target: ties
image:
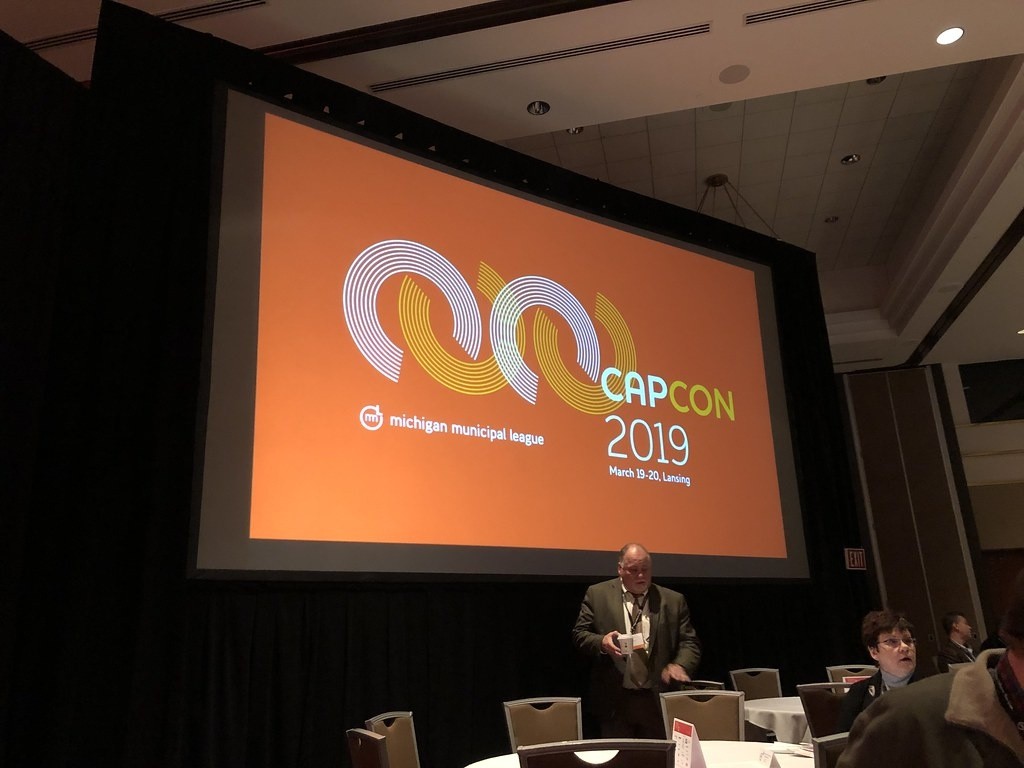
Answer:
[630,591,650,688]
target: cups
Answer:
[617,634,633,654]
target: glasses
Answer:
[877,637,917,647]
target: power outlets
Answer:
[928,633,934,642]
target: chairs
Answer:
[796,682,853,739]
[659,689,745,742]
[932,656,942,674]
[503,697,583,754]
[517,738,676,768]
[365,711,420,768]
[811,732,849,768]
[346,728,390,768]
[680,680,724,691]
[826,665,879,696]
[729,668,782,701]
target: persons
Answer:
[834,609,925,731]
[833,568,1024,768]
[936,612,986,673]
[571,540,700,739]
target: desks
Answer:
[463,739,816,768]
[743,696,812,744]
